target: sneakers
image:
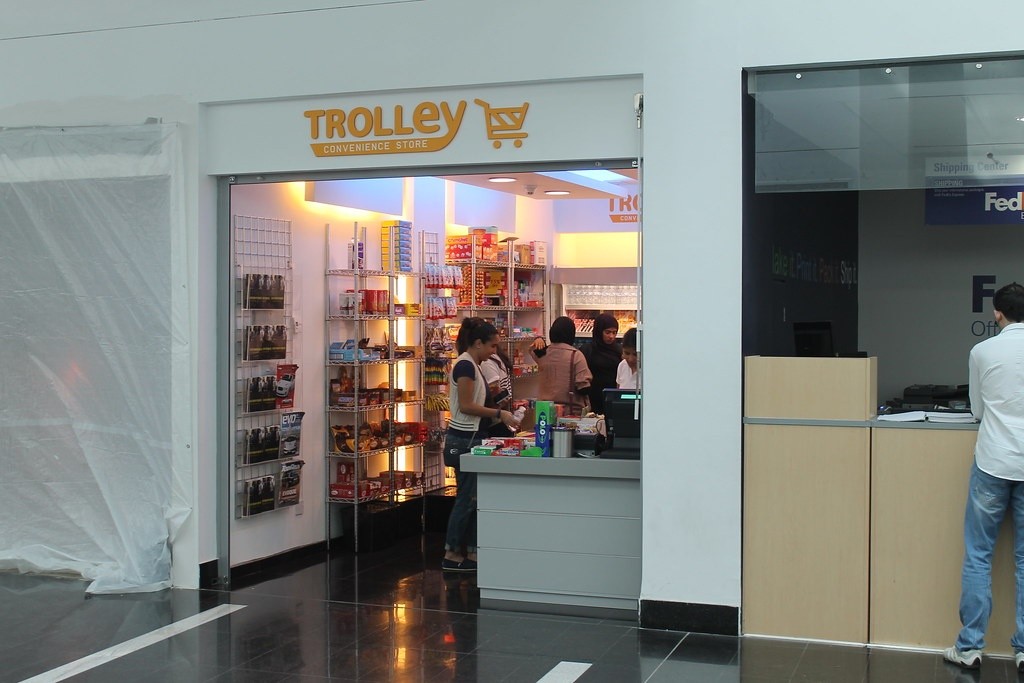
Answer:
[1016,652,1024,672]
[943,646,982,669]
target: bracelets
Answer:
[496,409,501,418]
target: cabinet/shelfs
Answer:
[565,303,638,339]
[320,222,428,562]
[417,229,511,496]
[512,243,547,435]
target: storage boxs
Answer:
[328,220,550,497]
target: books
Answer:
[877,411,976,423]
[243,273,306,517]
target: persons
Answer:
[941,282,1024,672]
[441,314,641,572]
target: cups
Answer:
[552,427,574,457]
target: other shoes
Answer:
[442,556,477,570]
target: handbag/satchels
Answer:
[443,443,469,467]
